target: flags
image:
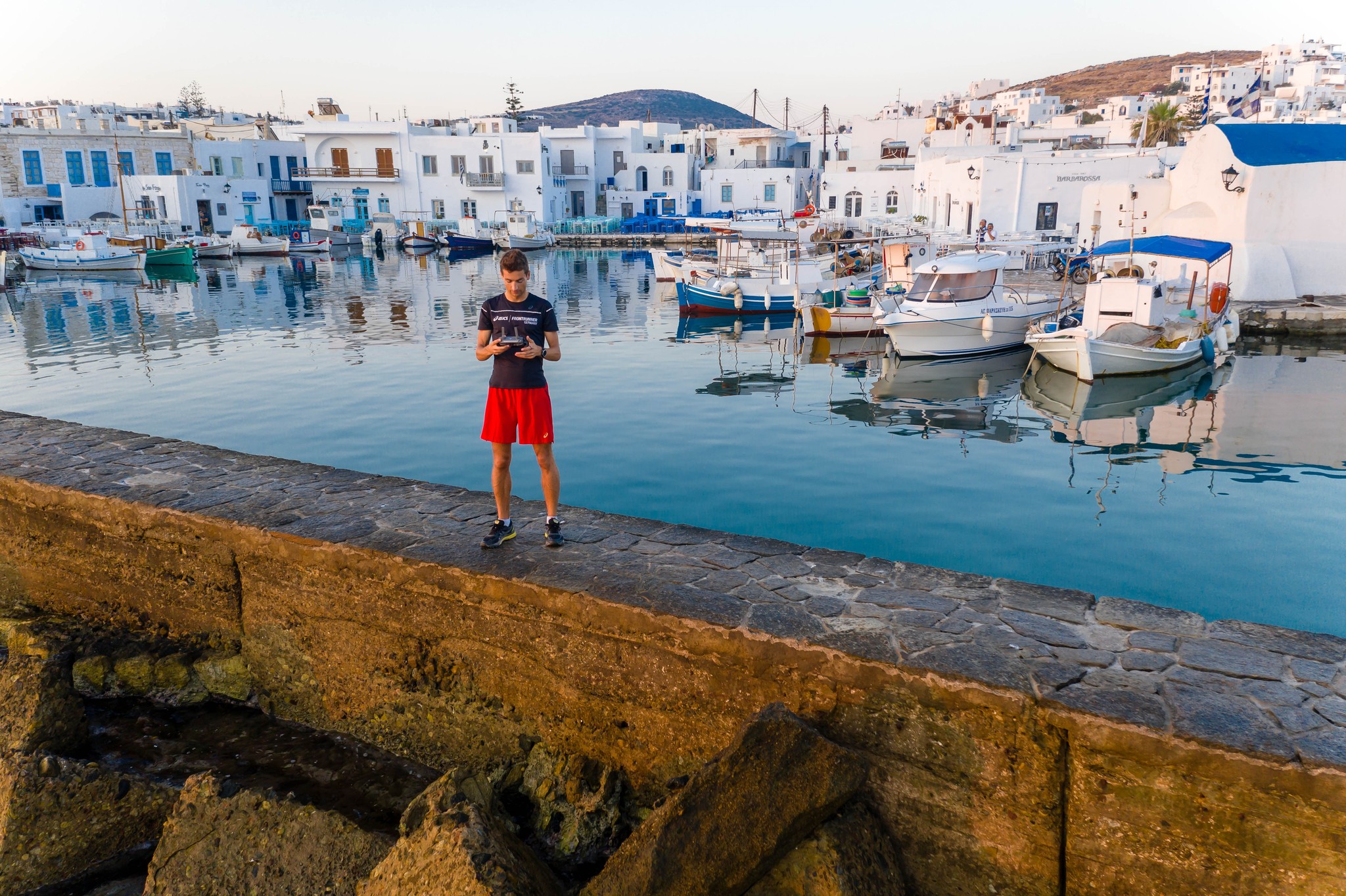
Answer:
[1226,73,1261,119]
[1200,69,1211,126]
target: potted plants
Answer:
[436,214,443,219]
[913,213,927,224]
[566,207,570,213]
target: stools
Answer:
[756,164,762,168]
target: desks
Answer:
[480,175,491,187]
[31,218,180,230]
[534,223,544,229]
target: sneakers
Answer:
[480,519,516,548]
[545,518,565,547]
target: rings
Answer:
[529,346,532,350]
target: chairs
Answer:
[618,185,625,191]
[555,249,717,269]
[253,219,311,236]
[820,215,1077,269]
[481,220,507,228]
[396,219,416,232]
[609,186,614,190]
[555,212,729,234]
[342,219,373,233]
[0,231,44,252]
[469,172,501,186]
[762,160,766,167]
[317,168,361,177]
[427,220,459,232]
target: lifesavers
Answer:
[805,205,815,215]
[180,236,188,238]
[464,216,474,218]
[76,242,84,250]
[293,232,300,239]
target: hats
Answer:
[199,211,203,213]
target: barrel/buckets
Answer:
[821,290,842,307]
[846,290,870,307]
[886,287,906,294]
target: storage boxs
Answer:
[247,231,262,241]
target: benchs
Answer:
[76,218,196,241]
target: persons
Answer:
[199,211,212,237]
[976,219,997,250]
[475,249,562,549]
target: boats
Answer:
[197,259,235,268]
[145,265,197,282]
[867,246,1077,361]
[1022,235,1241,385]
[493,197,552,252]
[648,178,887,318]
[799,337,894,364]
[444,216,496,250]
[399,211,435,247]
[796,233,938,338]
[233,256,293,269]
[674,317,804,344]
[868,354,1026,401]
[290,205,368,245]
[18,132,289,270]
[360,213,410,247]
[1018,362,1216,430]
[25,269,151,285]
[289,237,333,253]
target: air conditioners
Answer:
[182,168,193,176]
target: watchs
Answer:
[539,346,548,359]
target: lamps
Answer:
[380,193,384,197]
[223,182,232,194]
[483,140,488,152]
[650,196,669,200]
[689,196,691,200]
[536,185,567,200]
[967,165,980,180]
[854,187,855,189]
[203,189,206,193]
[887,209,895,214]
[892,184,926,193]
[1221,164,1245,193]
[726,174,790,183]
[259,197,261,201]
[932,265,938,273]
[613,196,628,200]
[822,180,827,190]
[334,192,337,197]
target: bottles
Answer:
[843,300,846,307]
[841,303,843,307]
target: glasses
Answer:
[988,223,993,225]
